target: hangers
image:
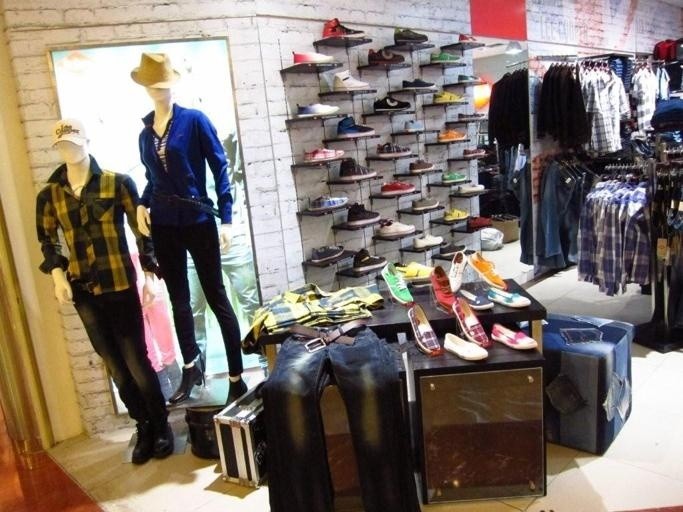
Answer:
[499,59,529,75]
[541,54,668,80]
[540,152,656,184]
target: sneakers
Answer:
[458,75,483,83]
[430,266,456,311]
[351,248,387,271]
[458,184,485,193]
[448,251,467,293]
[322,18,365,38]
[347,203,380,225]
[437,129,468,142]
[409,159,435,173]
[337,117,375,138]
[433,92,463,103]
[303,148,345,163]
[381,263,414,306]
[394,27,428,43]
[402,79,436,89]
[441,171,467,183]
[463,149,486,158]
[333,71,370,90]
[394,261,435,282]
[413,233,443,248]
[412,198,439,211]
[467,251,508,290]
[379,219,416,237]
[381,181,416,195]
[376,142,412,157]
[294,53,334,63]
[308,195,348,210]
[458,113,487,120]
[311,245,344,263]
[458,34,476,42]
[440,242,466,257]
[404,120,425,132]
[374,97,411,111]
[444,208,468,221]
[296,103,340,118]
[339,158,377,180]
[430,51,461,62]
[368,49,404,64]
[466,216,493,230]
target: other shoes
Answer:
[444,333,488,361]
[457,289,494,310]
[488,288,531,307]
[452,297,489,347]
[408,303,441,357]
[491,323,537,349]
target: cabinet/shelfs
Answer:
[263,340,410,504]
[405,335,553,505]
[258,276,550,472]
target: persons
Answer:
[123,213,182,404]
[185,160,268,386]
[129,53,248,407]
[34,117,175,466]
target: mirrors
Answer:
[468,32,535,283]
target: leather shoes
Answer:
[168,362,202,403]
[132,420,173,464]
[225,376,247,406]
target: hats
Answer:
[131,52,179,89]
[53,119,89,145]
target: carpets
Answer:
[44,226,682,512]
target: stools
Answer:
[530,311,634,457]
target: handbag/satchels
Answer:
[655,39,677,60]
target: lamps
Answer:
[503,40,525,56]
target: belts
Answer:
[291,319,366,352]
[152,191,221,217]
